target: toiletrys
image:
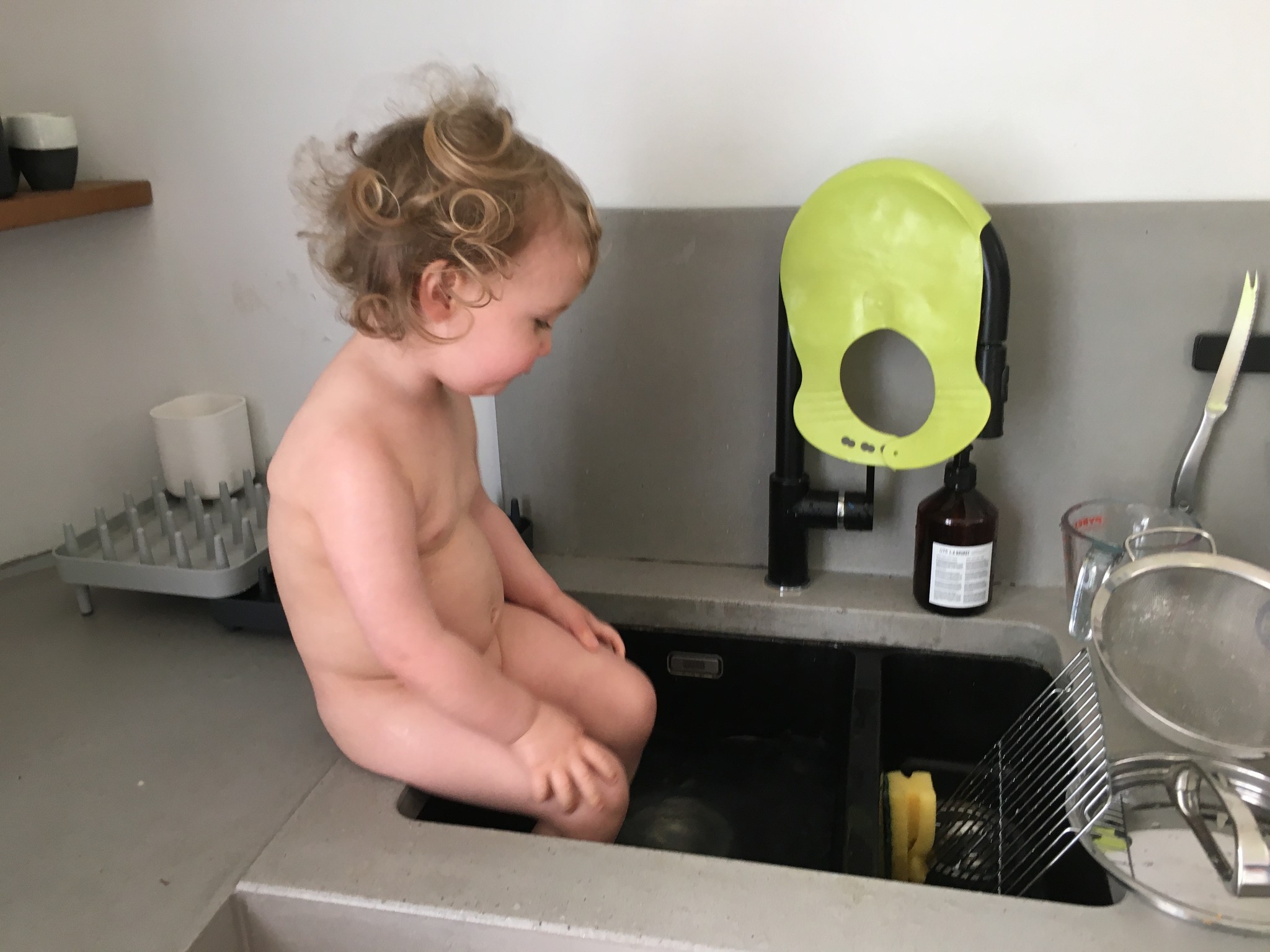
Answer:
[912,439,999,617]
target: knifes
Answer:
[1169,270,1262,518]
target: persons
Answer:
[264,59,660,845]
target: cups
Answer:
[1061,499,1203,639]
[150,392,256,500]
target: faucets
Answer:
[767,210,1013,595]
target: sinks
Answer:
[397,586,1137,907]
